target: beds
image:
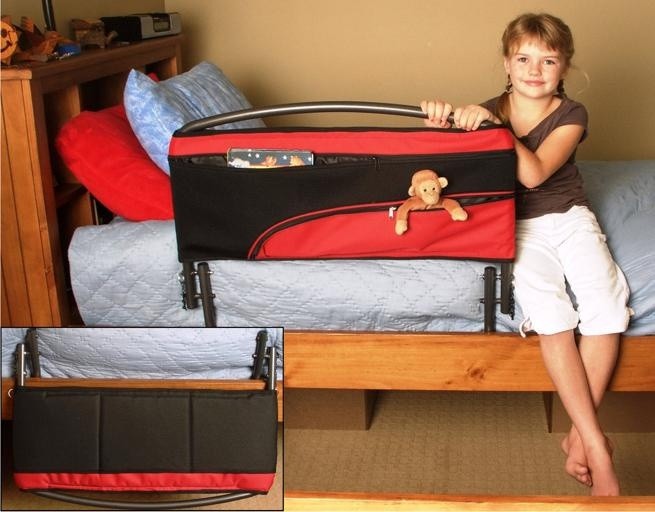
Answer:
[68,158,653,392]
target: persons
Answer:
[420,11,633,498]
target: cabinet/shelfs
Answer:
[0,32,189,326]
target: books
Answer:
[226,146,315,170]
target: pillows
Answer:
[58,60,266,220]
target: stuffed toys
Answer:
[393,168,469,236]
